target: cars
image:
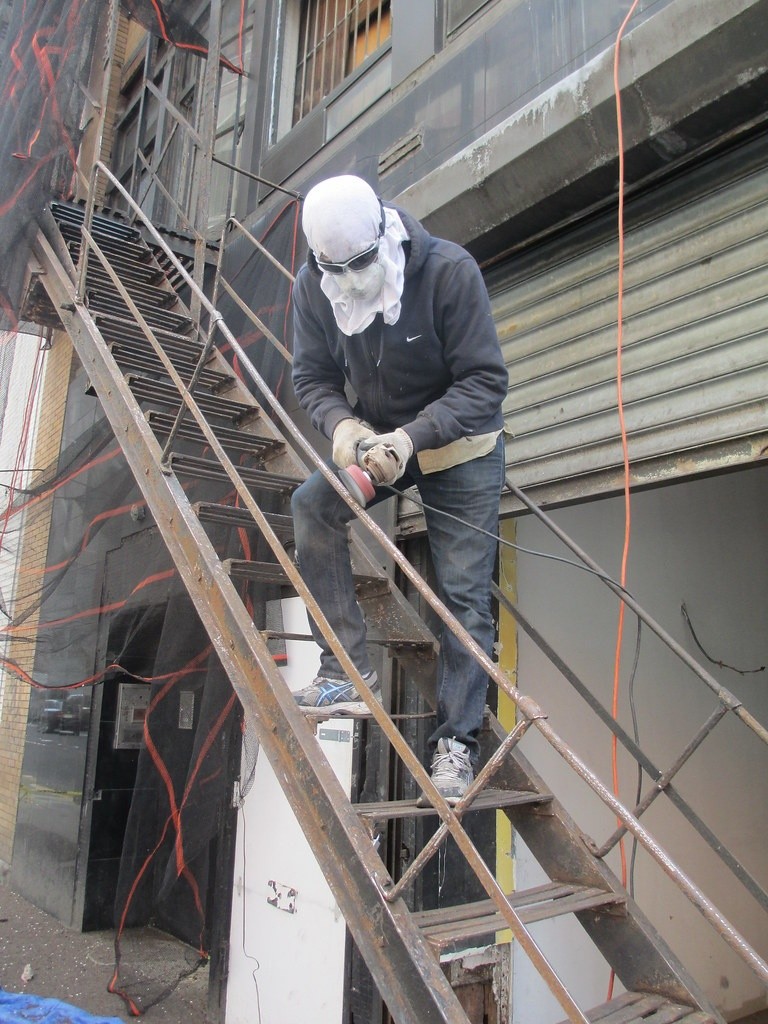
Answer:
[41,693,93,733]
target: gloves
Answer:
[332,418,376,469]
[359,428,414,485]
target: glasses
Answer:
[315,235,379,275]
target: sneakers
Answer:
[292,670,382,715]
[417,736,475,807]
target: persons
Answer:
[289,174,509,808]
